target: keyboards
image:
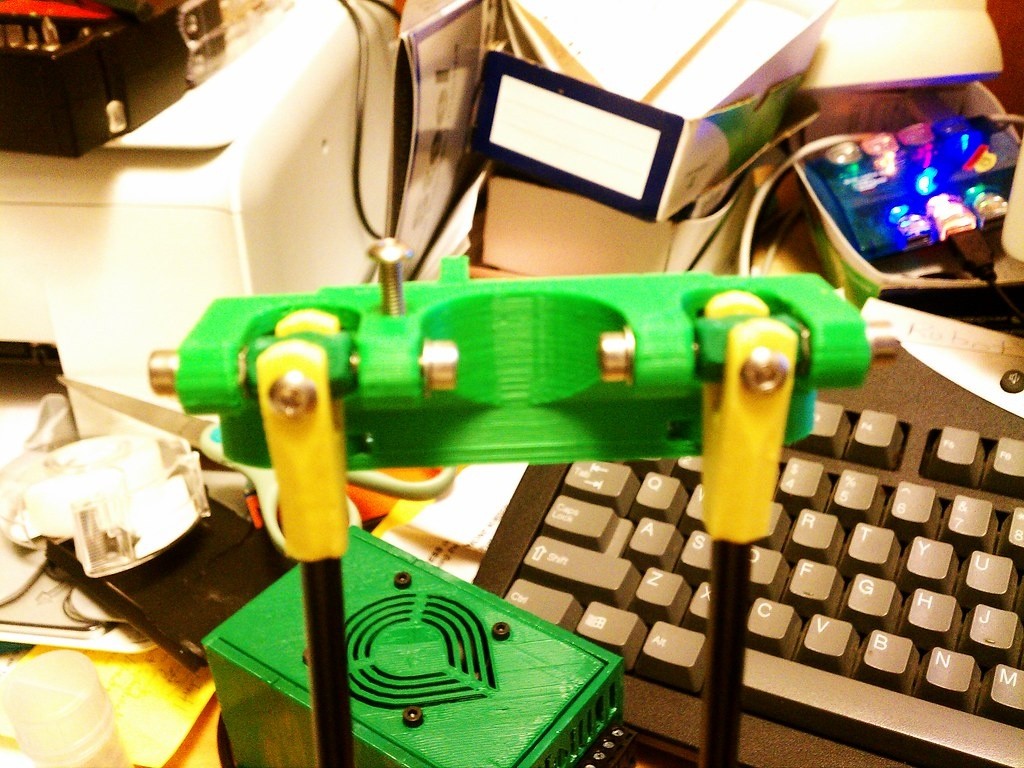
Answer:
[471,343,1024,768]
